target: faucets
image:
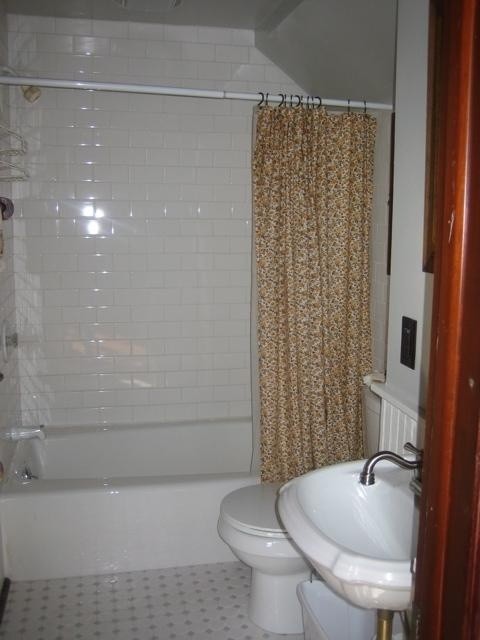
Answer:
[12,426,46,442]
[360,451,419,485]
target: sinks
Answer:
[278,458,417,611]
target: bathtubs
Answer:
[0,418,252,584]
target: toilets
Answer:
[217,483,322,635]
